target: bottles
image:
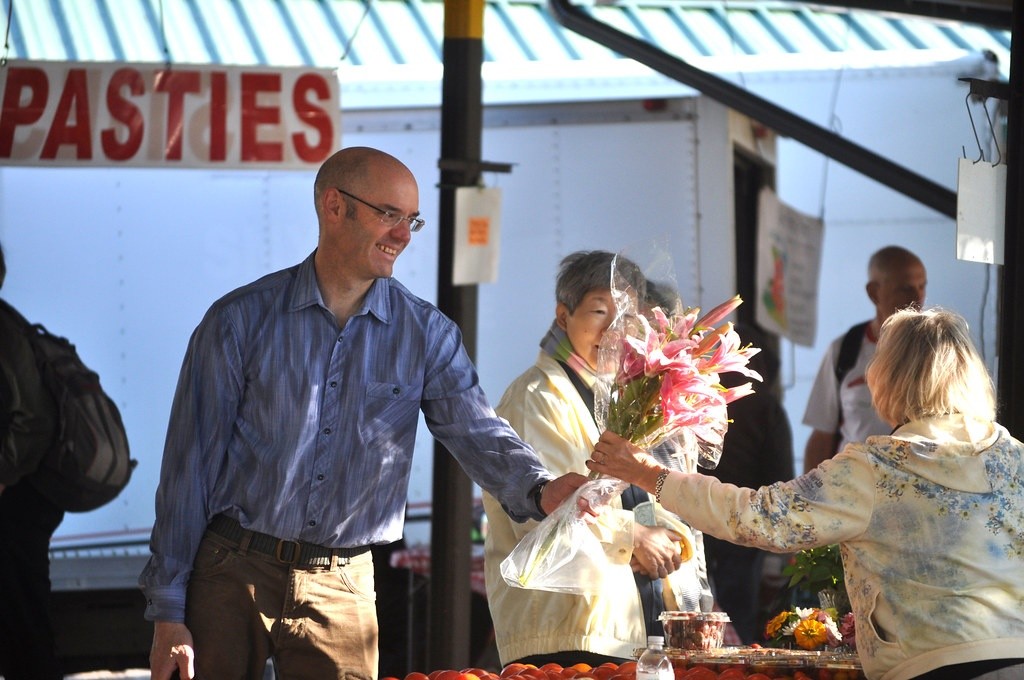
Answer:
[635,635,674,680]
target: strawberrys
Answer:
[663,612,725,650]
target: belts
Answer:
[207,514,376,564]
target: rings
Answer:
[599,453,607,464]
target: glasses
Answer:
[337,189,425,232]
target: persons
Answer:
[136,147,590,680]
[584,311,1023,680]
[694,324,796,647]
[641,280,716,617]
[479,250,694,669]
[801,244,926,475]
[1,242,65,680]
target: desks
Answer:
[632,645,863,671]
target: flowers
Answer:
[588,238,764,483]
[764,604,856,652]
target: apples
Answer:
[382,662,811,680]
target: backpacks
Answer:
[0,299,131,513]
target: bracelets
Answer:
[526,479,552,519]
[655,468,670,502]
